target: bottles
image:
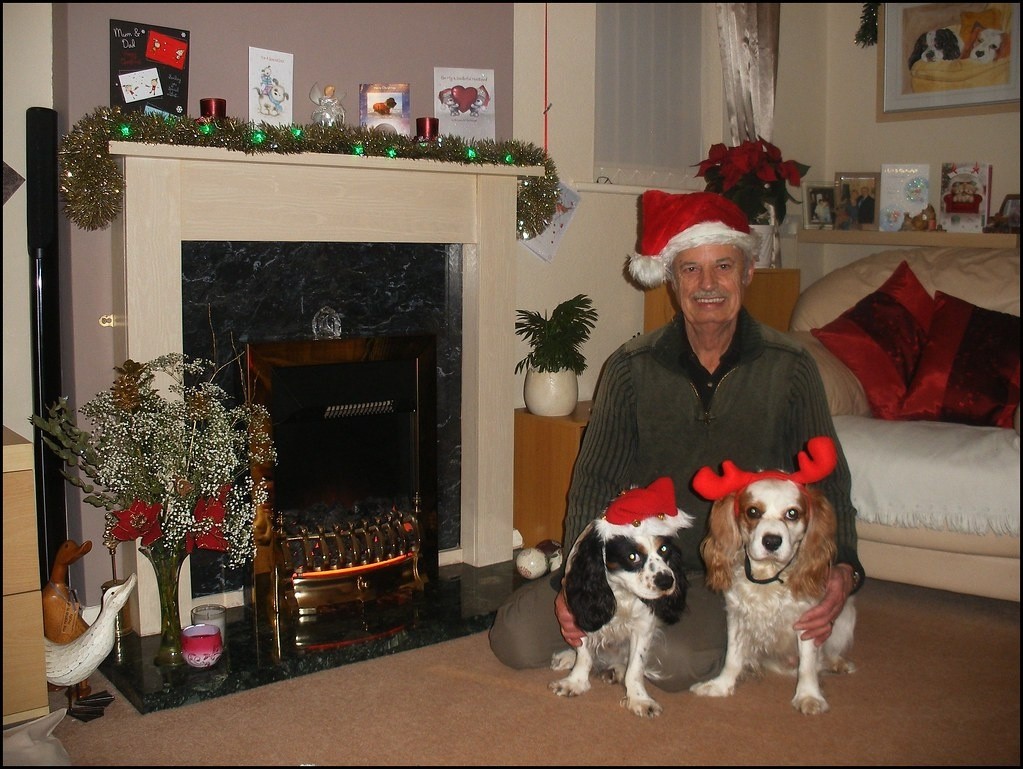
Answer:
[416,118,439,140]
[310,86,345,126]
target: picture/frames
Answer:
[359,82,410,135]
[875,3,1020,122]
[801,181,837,229]
[836,172,881,232]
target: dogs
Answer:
[547,485,696,716]
[691,467,861,716]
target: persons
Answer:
[811,195,834,223]
[486,189,866,671]
[311,81,345,121]
[856,187,874,223]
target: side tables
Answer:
[641,268,802,337]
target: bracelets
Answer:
[854,569,860,588]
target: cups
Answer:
[191,604,226,652]
[180,624,223,668]
[200,98,226,118]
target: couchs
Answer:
[787,248,1021,603]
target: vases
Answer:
[747,223,773,268]
[136,552,187,670]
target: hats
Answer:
[627,189,753,288]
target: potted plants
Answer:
[518,291,595,420]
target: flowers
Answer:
[27,358,269,549]
[692,140,808,225]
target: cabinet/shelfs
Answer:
[514,401,591,553]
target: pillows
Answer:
[902,291,1021,431]
[810,261,932,421]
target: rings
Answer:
[831,622,833,625]
